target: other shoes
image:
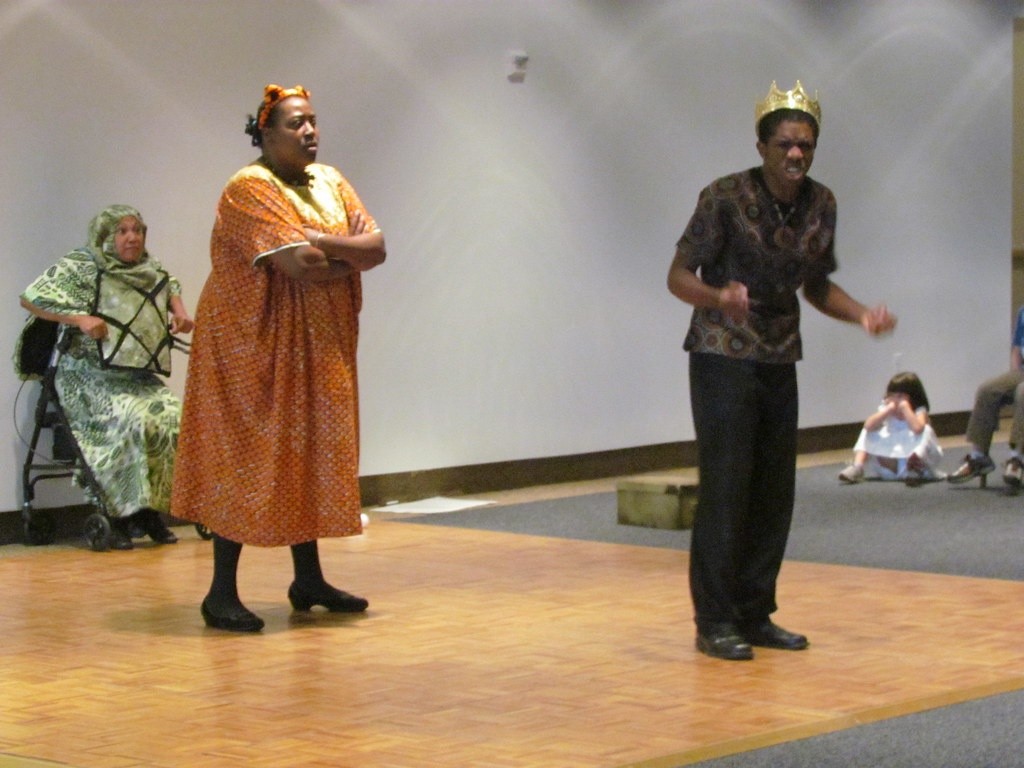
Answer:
[288,579,368,613]
[111,530,132,549]
[139,514,178,544]
[1004,457,1024,486]
[200,601,264,630]
[948,452,996,484]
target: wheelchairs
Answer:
[20,322,214,551]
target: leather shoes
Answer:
[743,617,809,649]
[696,618,753,659]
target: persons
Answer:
[169,84,388,631]
[666,78,897,660]
[19,204,194,550]
[947,306,1024,484]
[838,371,947,487]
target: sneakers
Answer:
[904,453,926,486]
[839,466,864,482]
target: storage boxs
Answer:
[614,476,699,531]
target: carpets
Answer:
[385,442,1024,768]
